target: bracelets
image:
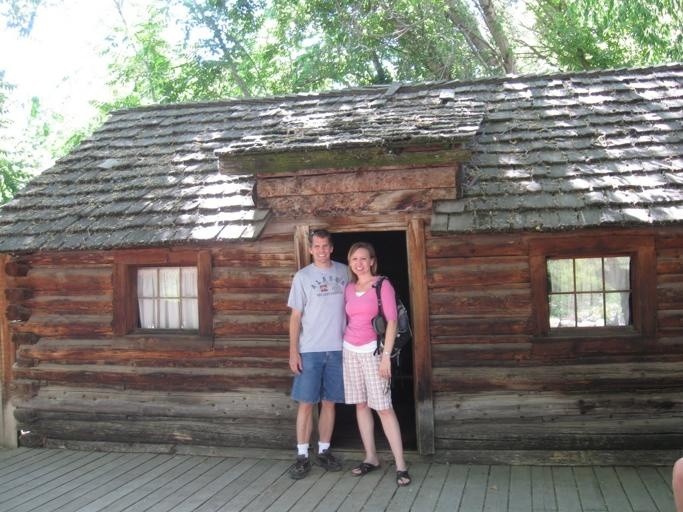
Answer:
[383,352,390,355]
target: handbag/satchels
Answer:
[370,277,414,352]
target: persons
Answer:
[342,241,411,486]
[287,230,354,479]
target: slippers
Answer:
[395,469,412,488]
[350,461,381,477]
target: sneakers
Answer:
[314,449,343,473]
[289,452,312,480]
